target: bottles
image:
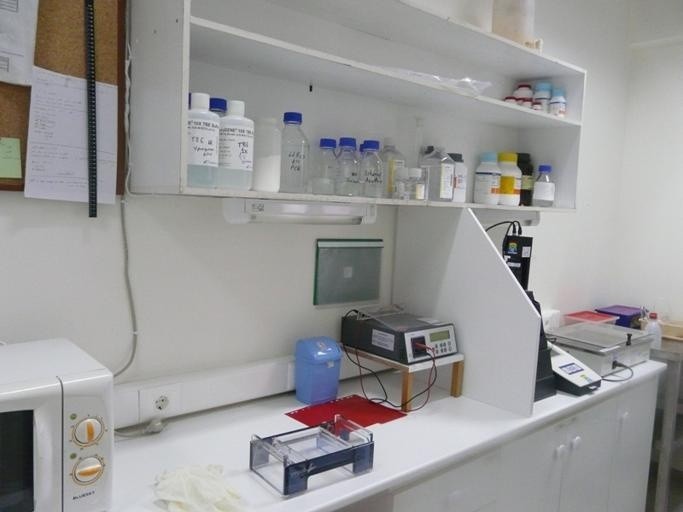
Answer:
[498,153,521,206]
[254,116,283,193]
[280,111,309,194]
[312,132,468,202]
[517,154,533,205]
[647,313,661,350]
[500,82,566,117]
[533,165,557,206]
[475,152,500,205]
[188,86,255,188]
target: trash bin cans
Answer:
[294,335,343,406]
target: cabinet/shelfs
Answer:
[501,396,609,512]
[348,445,500,511]
[610,378,658,511]
[130,0,588,212]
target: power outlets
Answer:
[139,382,183,422]
